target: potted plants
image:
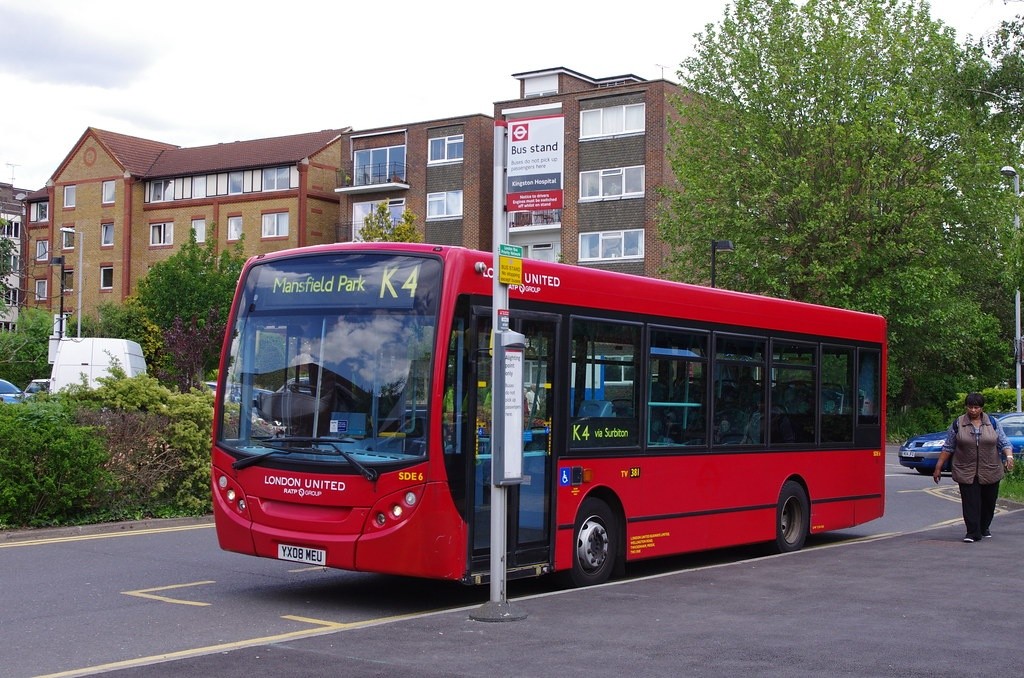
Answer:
[344,175,352,187]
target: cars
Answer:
[898,411,1024,477]
[0,379,30,407]
[22,378,52,402]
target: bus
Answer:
[209,238,889,591]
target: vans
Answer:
[48,336,147,396]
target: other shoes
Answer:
[982,531,992,538]
[964,536,976,542]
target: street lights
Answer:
[711,238,735,288]
[59,227,84,338]
[48,254,66,341]
[1001,164,1022,412]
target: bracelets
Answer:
[1006,455,1015,460]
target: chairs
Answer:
[647,373,845,445]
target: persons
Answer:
[522,383,542,416]
[934,393,1015,543]
